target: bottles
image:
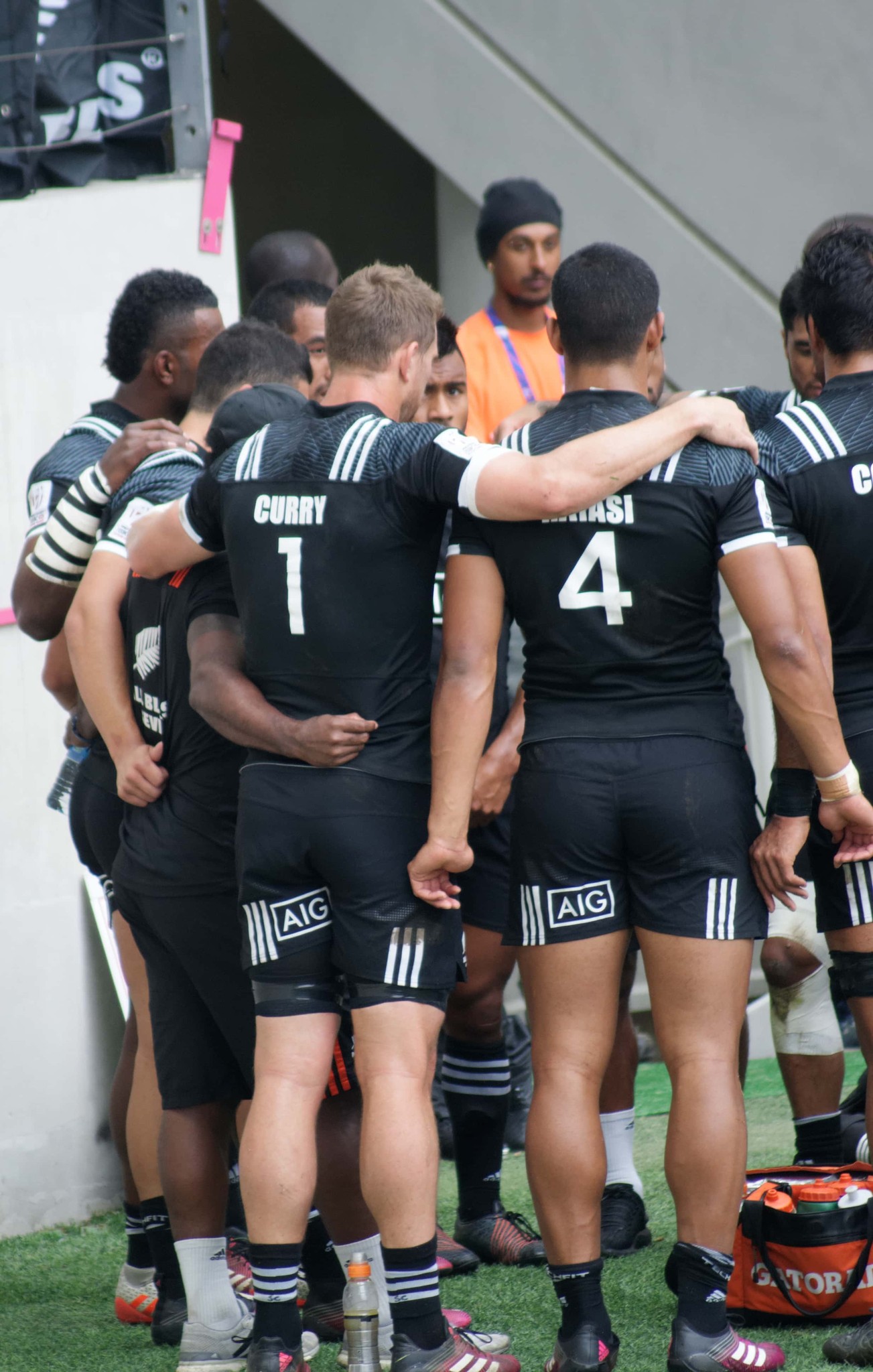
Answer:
[46,743,87,814]
[741,1173,873,1214]
[342,1252,383,1372]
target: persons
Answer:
[12,177,873,1372]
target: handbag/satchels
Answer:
[726,1161,873,1323]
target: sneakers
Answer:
[435,1224,480,1272]
[337,1323,511,1368]
[390,1316,521,1372]
[305,1298,344,1340]
[435,1255,454,1276]
[243,1335,311,1372]
[226,1238,253,1294]
[505,1014,534,1151]
[667,1316,784,1372]
[543,1321,620,1372]
[178,1299,321,1372]
[453,1201,547,1267]
[822,1315,873,1365]
[152,1296,258,1345]
[116,1271,159,1325]
[430,1030,458,1160]
[600,1182,651,1254]
[441,1308,472,1332]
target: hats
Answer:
[205,383,308,450]
[476,177,562,268]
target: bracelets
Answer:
[814,760,862,802]
